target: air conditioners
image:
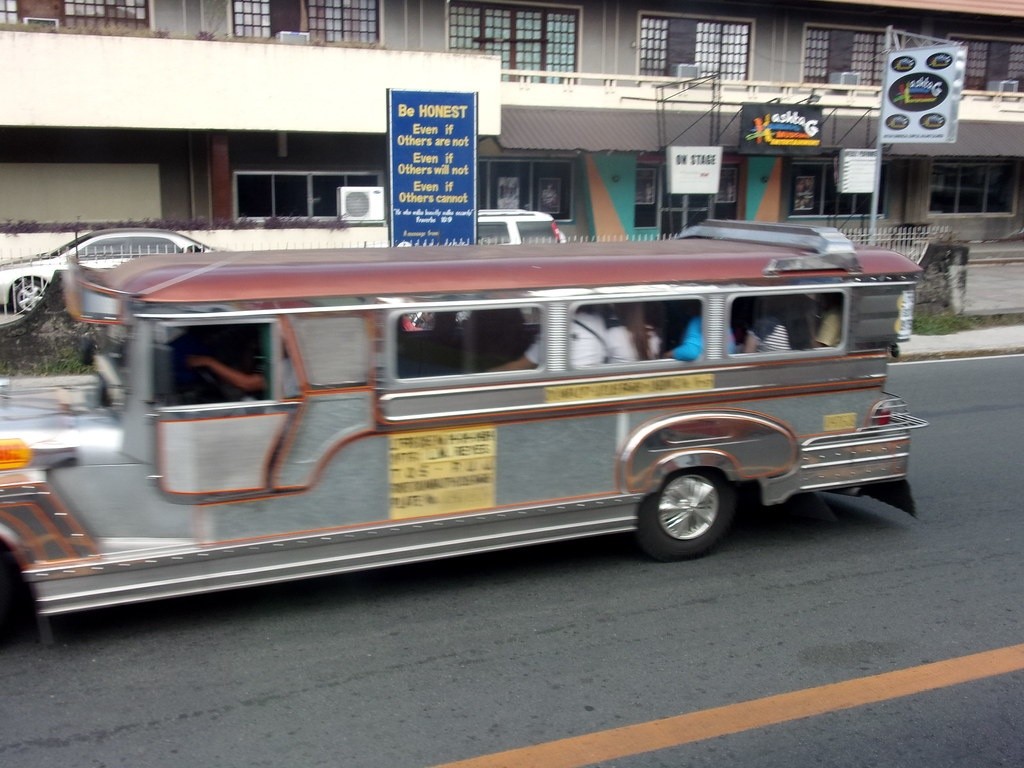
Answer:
[828,72,861,85]
[336,186,384,221]
[989,81,1019,93]
[275,31,310,43]
[23,16,59,28]
[670,63,700,77]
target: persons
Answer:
[182,323,270,404]
[474,293,844,372]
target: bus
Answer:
[0,218,933,650]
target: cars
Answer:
[0,228,219,313]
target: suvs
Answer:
[476,209,569,248]
